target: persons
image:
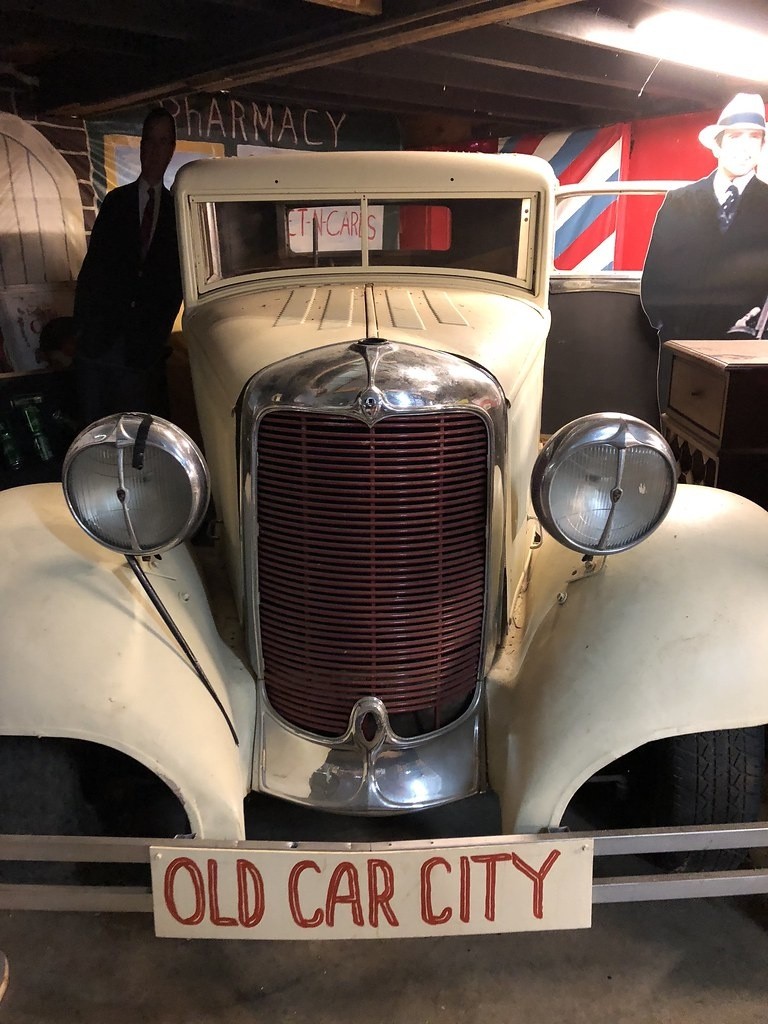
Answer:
[73,109,183,421]
[640,92,768,436]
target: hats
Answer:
[698,92,768,150]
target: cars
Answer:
[0,147,768,940]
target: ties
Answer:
[720,186,739,229]
[139,182,156,246]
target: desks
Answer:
[661,340,768,497]
